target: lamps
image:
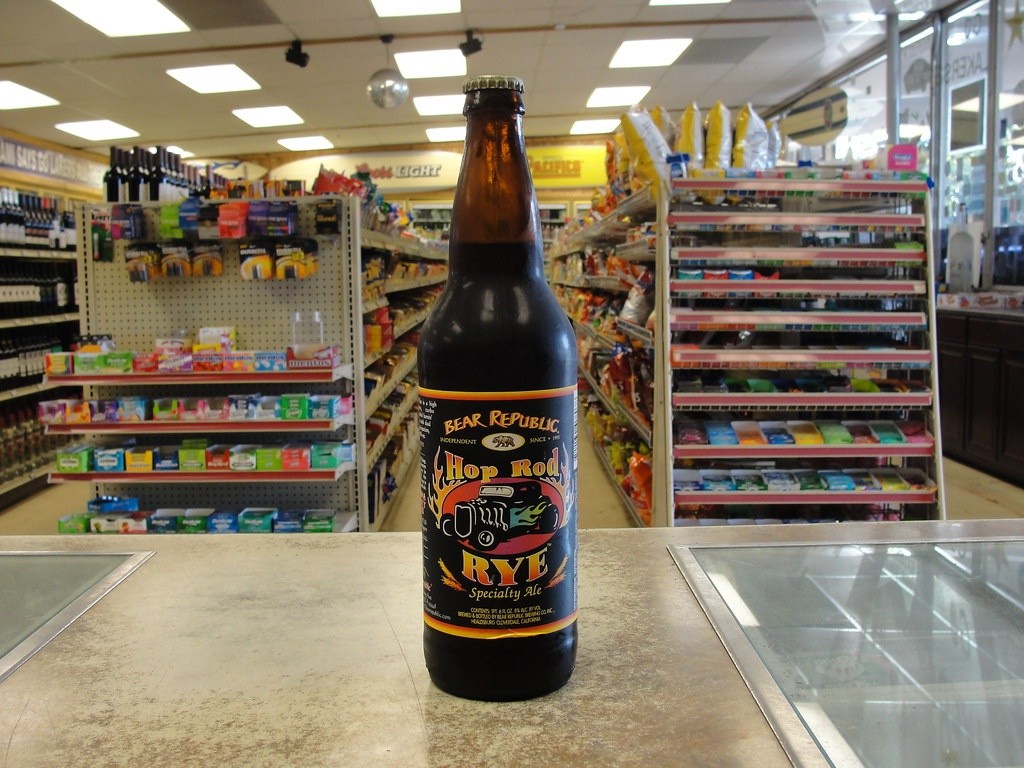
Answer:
[286,40,309,68]
[458,29,485,57]
[366,35,408,111]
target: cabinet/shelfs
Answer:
[405,204,559,280]
[936,311,1024,489]
[79,197,447,537]
[0,248,84,504]
[0,196,79,502]
[559,179,946,530]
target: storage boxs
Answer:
[365,263,447,521]
[38,328,356,537]
[671,270,939,526]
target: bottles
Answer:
[0,143,228,484]
[417,74,577,701]
